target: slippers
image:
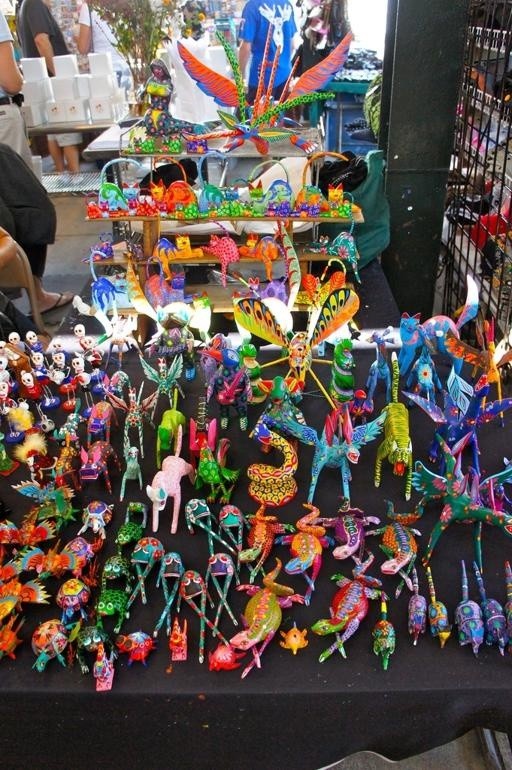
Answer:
[28,291,74,316]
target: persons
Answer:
[165,1,223,123]
[237,0,298,113]
[14,0,85,187]
[71,0,135,171]
[0,8,35,177]
[292,0,353,129]
[1,140,76,315]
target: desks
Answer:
[82,120,326,248]
[31,121,114,132]
[2,343,509,764]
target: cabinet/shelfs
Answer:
[82,207,363,348]
